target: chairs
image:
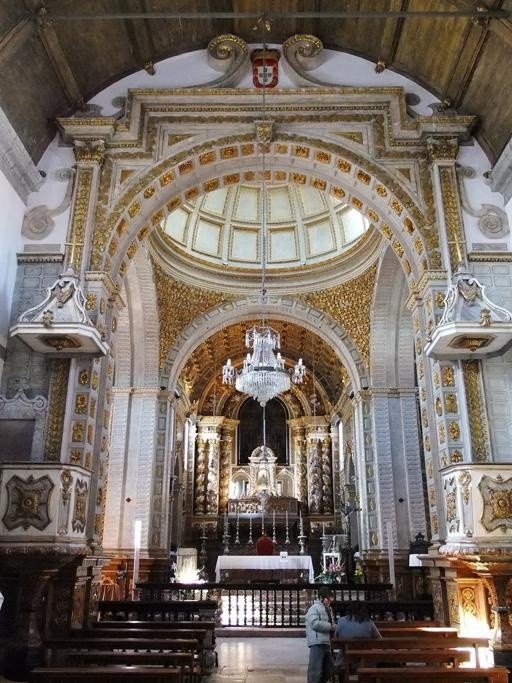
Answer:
[255,536,275,556]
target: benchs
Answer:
[28,620,219,682]
[334,622,509,683]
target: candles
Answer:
[202,509,327,538]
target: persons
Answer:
[305,585,337,683]
[333,601,383,683]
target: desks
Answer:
[215,555,315,585]
[98,600,217,622]
[331,598,435,621]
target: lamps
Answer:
[223,144,306,407]
